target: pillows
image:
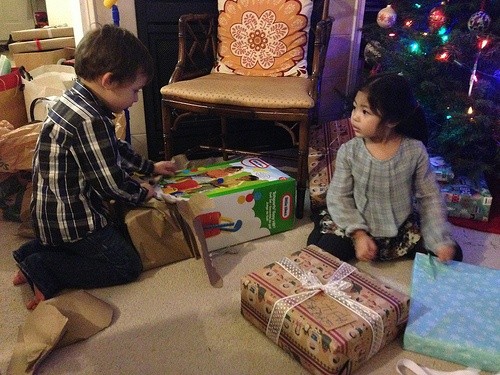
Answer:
[217,0,313,79]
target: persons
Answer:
[11,21,180,312]
[306,73,464,266]
[163,165,243,193]
[169,174,260,197]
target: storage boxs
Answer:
[402,253,500,374]
[12,26,73,41]
[10,36,75,54]
[434,159,492,221]
[123,159,297,273]
[309,119,356,212]
[10,48,68,72]
[240,245,410,375]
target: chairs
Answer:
[159,0,334,222]
